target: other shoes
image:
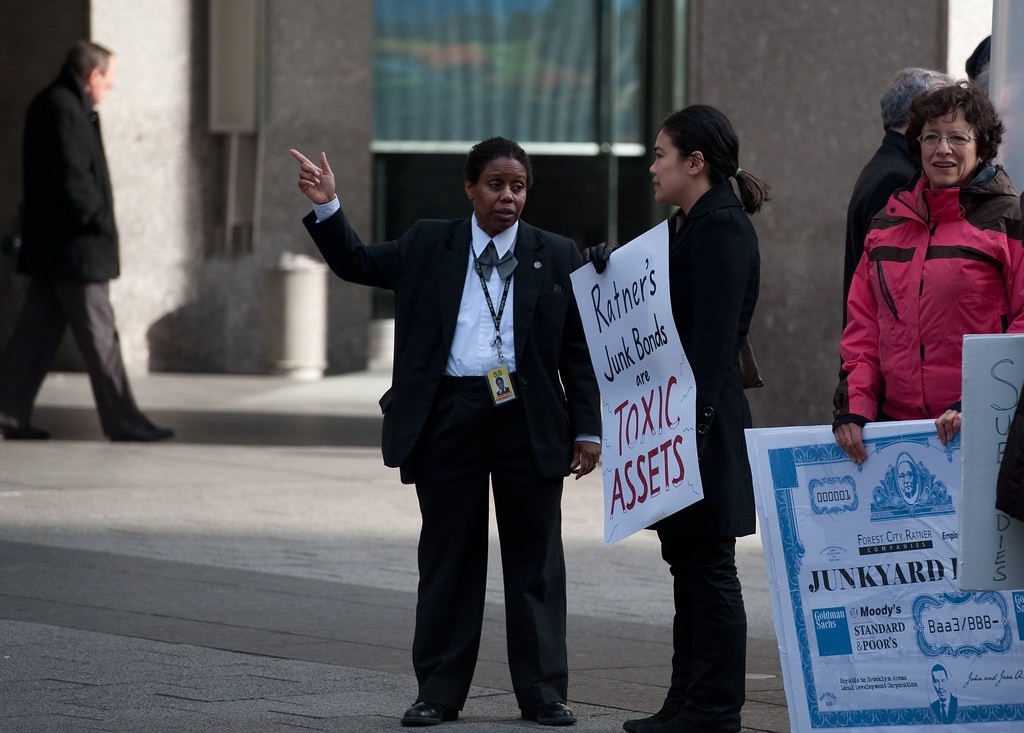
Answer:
[622,711,741,733]
[108,423,174,442]
[3,426,51,439]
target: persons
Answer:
[996,189,1024,522]
[0,39,175,443]
[831,34,1023,466]
[288,136,601,727]
[583,107,774,733]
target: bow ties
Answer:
[473,241,518,282]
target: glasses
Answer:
[917,131,977,146]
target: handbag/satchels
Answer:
[737,335,764,388]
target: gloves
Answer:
[583,242,619,274]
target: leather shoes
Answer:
[401,701,458,726]
[521,702,577,724]
